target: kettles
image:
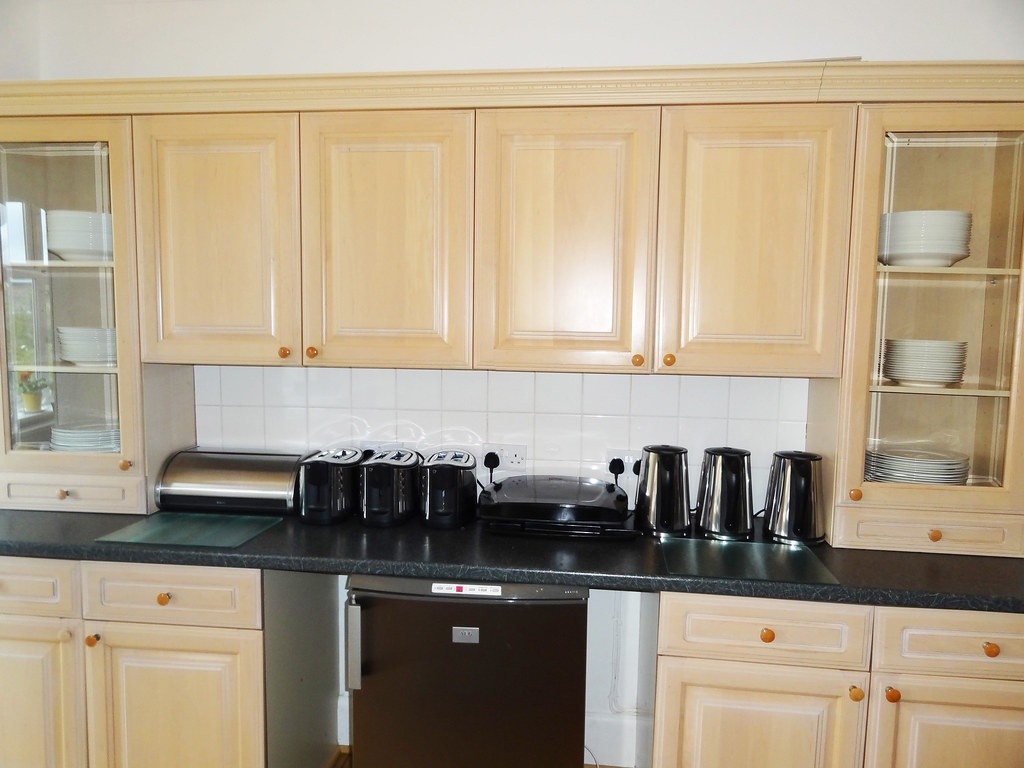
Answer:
[696,446,754,541]
[762,452,826,547]
[634,445,690,537]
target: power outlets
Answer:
[481,443,528,472]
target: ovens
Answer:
[345,583,589,768]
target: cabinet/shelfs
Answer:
[652,591,1024,768]
[0,557,339,768]
[0,58,1024,559]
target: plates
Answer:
[14,422,121,452]
[46,210,112,261]
[877,210,971,266]
[864,444,970,486]
[58,327,116,367]
[878,337,968,387]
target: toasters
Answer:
[422,449,476,528]
[360,448,425,528]
[299,446,363,524]
